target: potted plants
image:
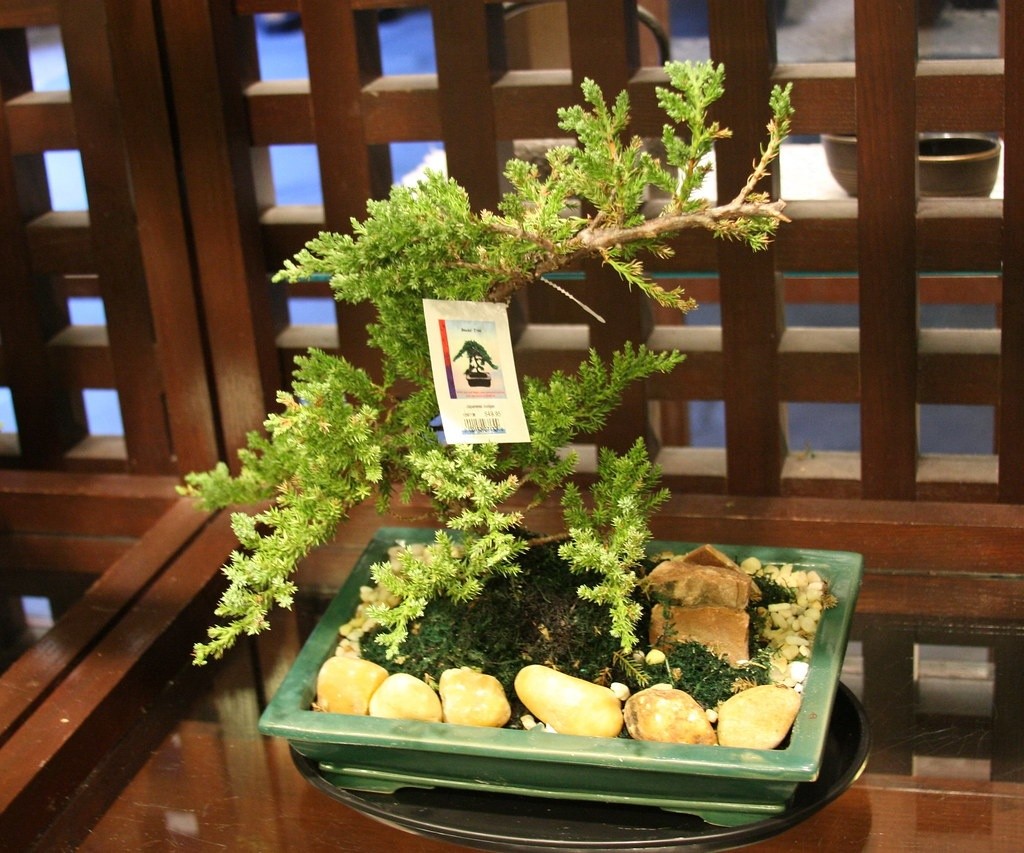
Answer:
[170,59,865,825]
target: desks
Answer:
[395,142,1005,453]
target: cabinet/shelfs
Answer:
[0,0,1024,853]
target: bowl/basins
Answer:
[819,133,1001,197]
